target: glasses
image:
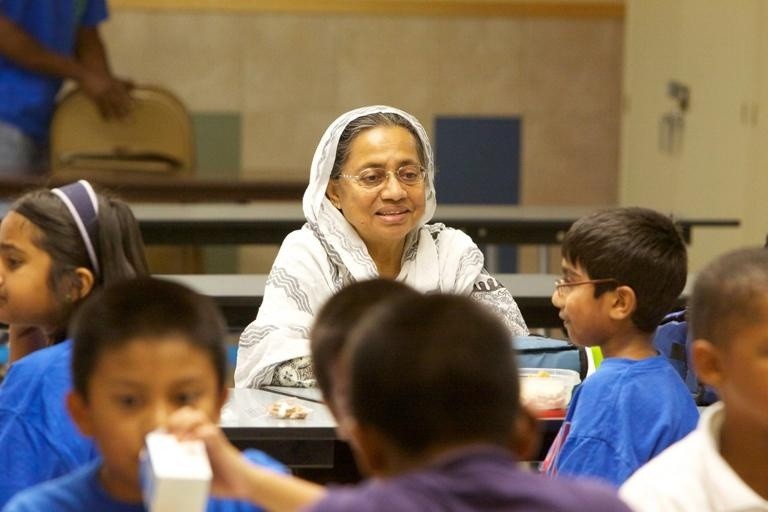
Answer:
[554,275,621,299]
[337,163,429,191]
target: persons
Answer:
[1,1,137,189]
[234,106,531,389]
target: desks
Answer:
[217,386,341,443]
[125,202,306,245]
[427,205,591,244]
[151,273,268,308]
[491,274,568,326]
[528,409,569,429]
[653,205,742,244]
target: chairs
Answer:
[44,80,200,278]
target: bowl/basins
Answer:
[519,367,581,412]
